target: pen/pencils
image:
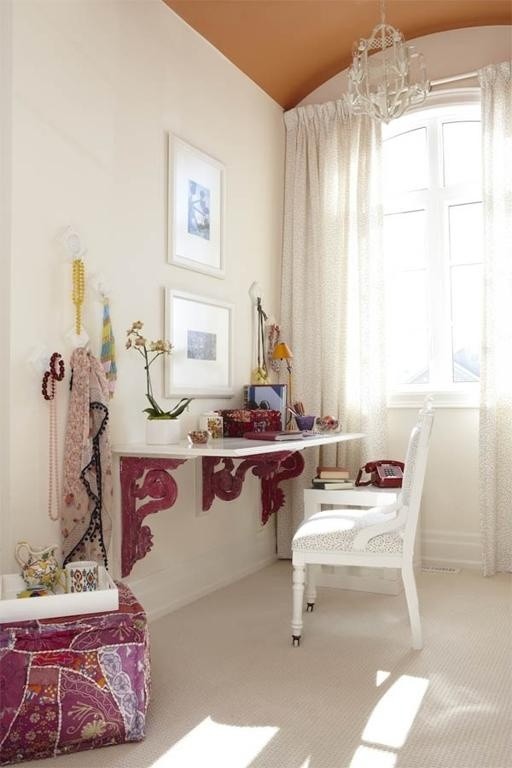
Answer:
[288,402,306,416]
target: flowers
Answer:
[290,408,435,653]
[125,320,196,421]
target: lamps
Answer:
[271,342,294,430]
[342,0,432,126]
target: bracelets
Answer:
[49,351,64,381]
[39,369,56,401]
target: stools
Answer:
[0,577,152,767]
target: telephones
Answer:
[365,458,404,488]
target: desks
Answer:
[111,430,368,578]
[303,480,423,597]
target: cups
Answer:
[207,416,223,438]
[65,560,98,595]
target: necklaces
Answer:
[47,357,62,522]
[72,255,86,334]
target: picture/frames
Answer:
[165,130,227,281]
[163,287,237,400]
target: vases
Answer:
[145,418,183,446]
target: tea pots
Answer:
[15,541,59,592]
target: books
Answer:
[313,475,345,483]
[244,431,304,440]
[312,479,355,490]
[317,466,350,479]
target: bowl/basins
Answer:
[316,419,340,433]
[295,416,315,429]
[188,431,211,443]
